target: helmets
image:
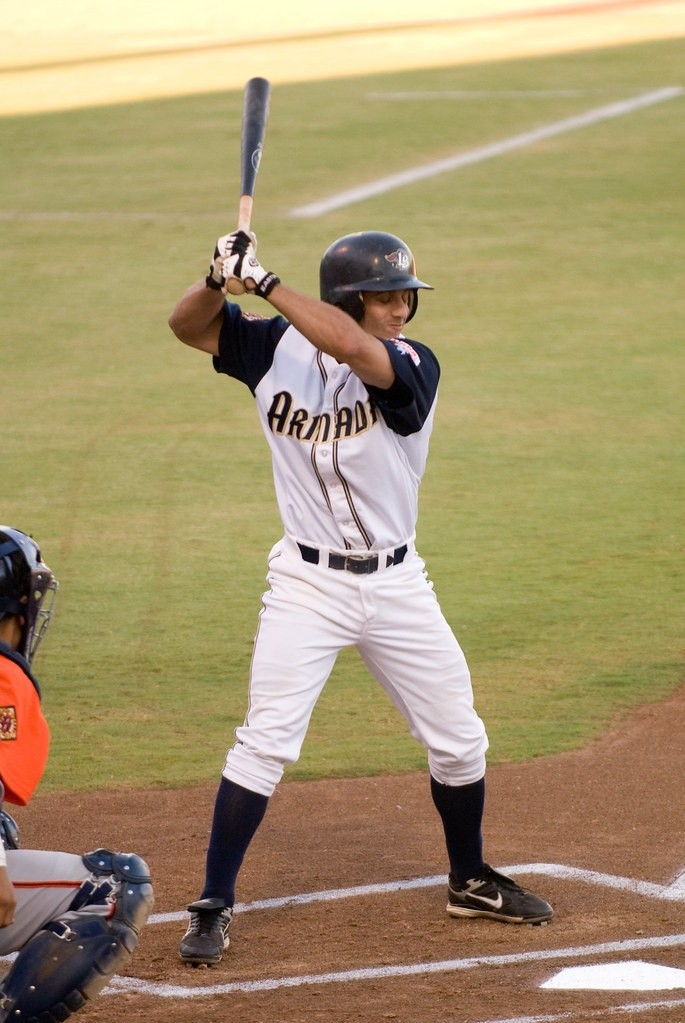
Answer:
[0,525,59,668]
[319,231,435,329]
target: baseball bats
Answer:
[217,78,268,297]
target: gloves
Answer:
[204,230,257,290]
[220,252,280,300]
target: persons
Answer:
[0,526,153,1023]
[169,231,556,965]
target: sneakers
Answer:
[446,864,554,922]
[178,898,233,963]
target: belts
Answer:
[296,542,407,574]
